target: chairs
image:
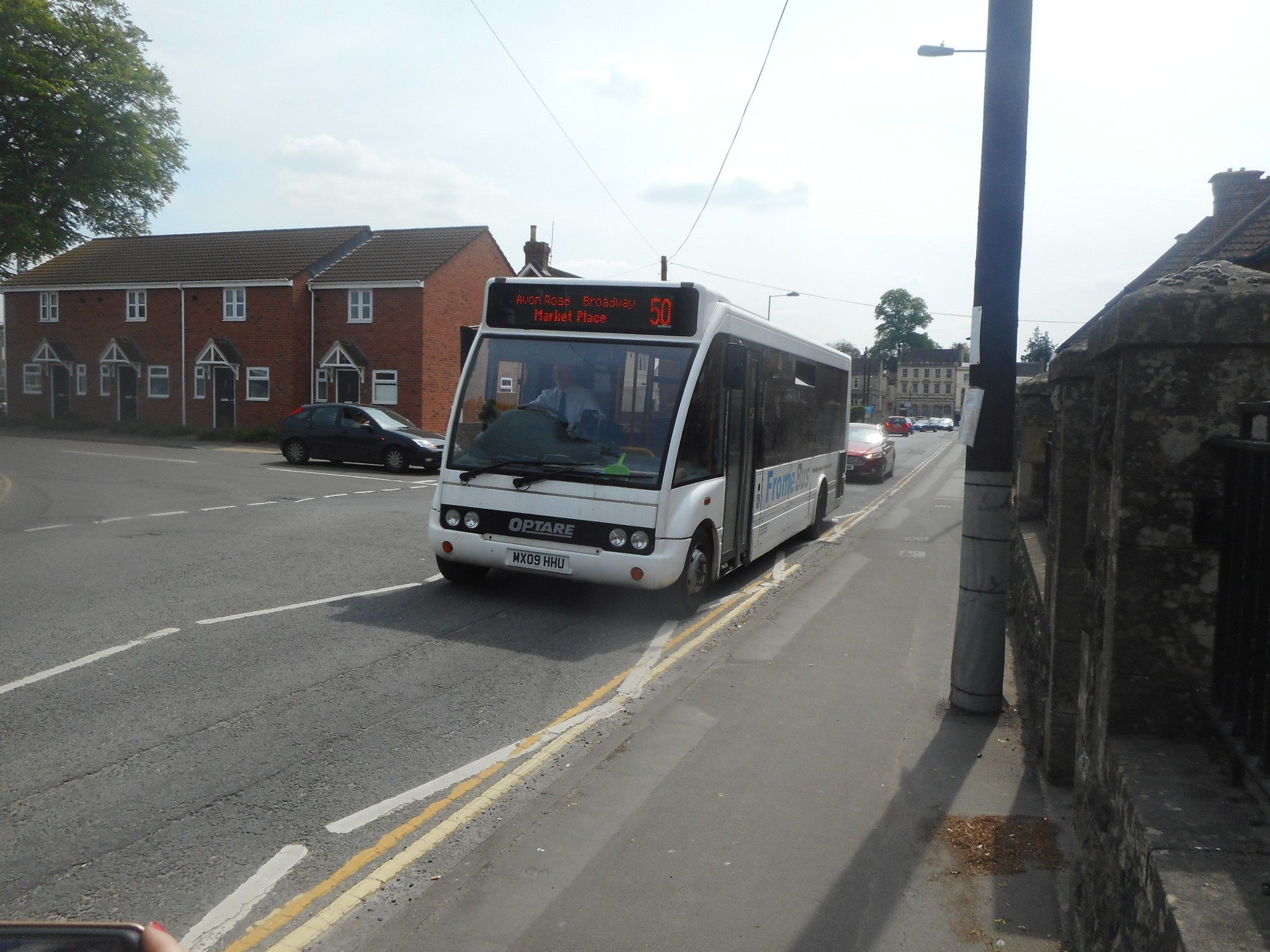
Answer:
[344,413,354,428]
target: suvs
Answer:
[846,423,896,483]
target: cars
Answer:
[278,402,464,473]
[886,415,954,437]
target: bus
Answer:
[427,276,853,621]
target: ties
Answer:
[558,392,567,415]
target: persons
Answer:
[141,921,184,952]
[352,413,370,428]
[517,358,606,441]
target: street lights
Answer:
[767,291,800,321]
